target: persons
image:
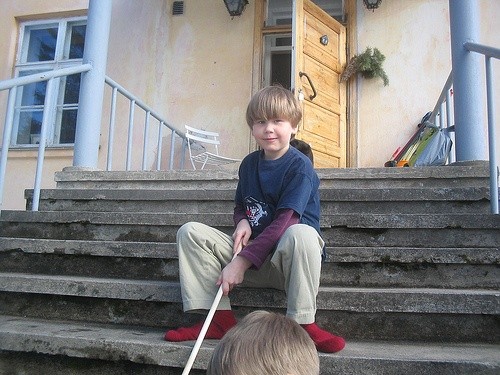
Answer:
[164,86,347,353]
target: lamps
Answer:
[223,0,249,16]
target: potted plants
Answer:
[342,47,389,87]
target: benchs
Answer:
[183,126,243,171]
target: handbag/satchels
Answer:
[408,129,453,166]
